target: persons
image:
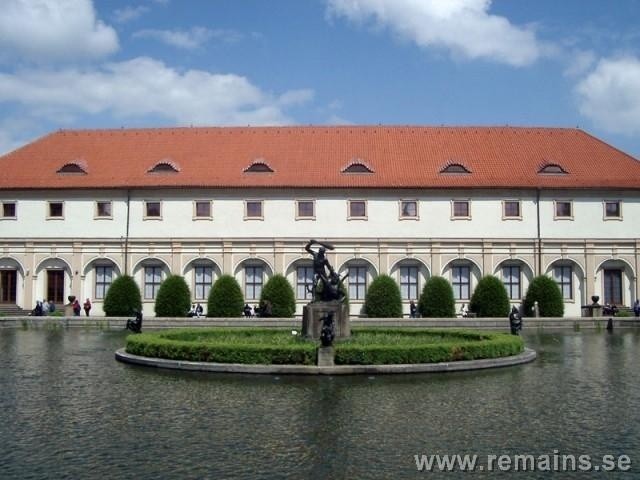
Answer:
[634,299,640,316]
[604,303,611,315]
[195,304,203,318]
[243,304,252,318]
[187,305,196,317]
[409,301,416,317]
[612,303,620,316]
[124,308,143,334]
[461,304,468,318]
[318,310,336,348]
[318,273,349,299]
[305,240,336,304]
[31,298,92,316]
